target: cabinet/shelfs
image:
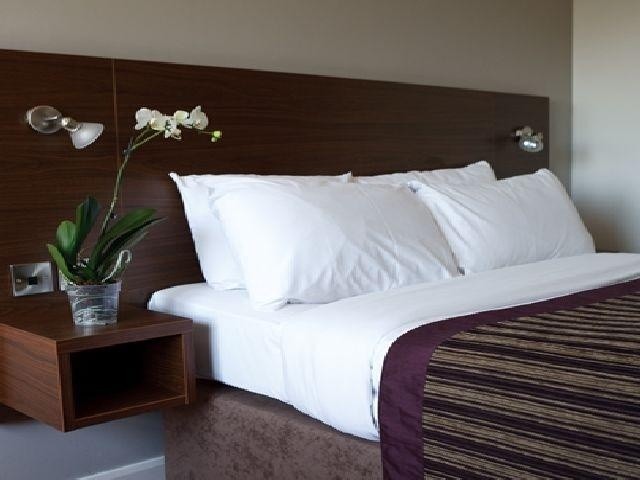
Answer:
[0,303,201,433]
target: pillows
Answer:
[354,161,499,193]
[406,168,597,273]
[202,181,460,309]
[169,171,359,290]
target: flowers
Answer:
[46,104,225,320]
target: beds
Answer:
[149,284,640,477]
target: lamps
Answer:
[26,107,104,150]
[515,126,544,153]
